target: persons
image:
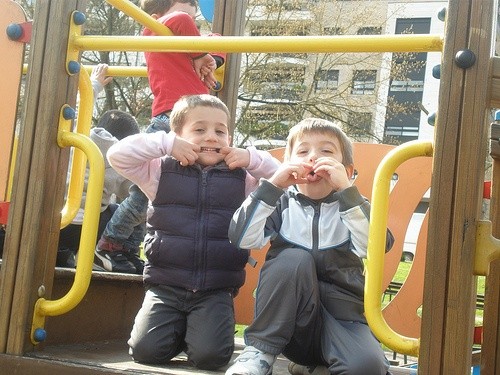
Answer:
[226,117,395,375]
[56,64,140,268]
[92,0,225,274]
[106,95,280,371]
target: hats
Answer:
[96,110,140,140]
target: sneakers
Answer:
[130,249,147,274]
[225,345,277,375]
[288,360,329,375]
[92,249,137,273]
